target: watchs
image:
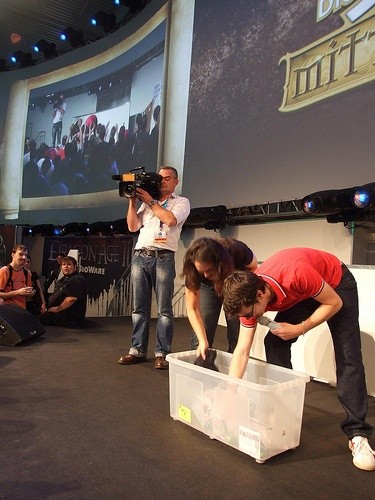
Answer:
[41,301,47,305]
[148,200,157,207]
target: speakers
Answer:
[0,303,47,346]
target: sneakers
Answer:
[348,436,375,470]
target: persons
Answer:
[0,244,36,310]
[42,256,87,327]
[23,94,161,198]
[25,256,47,314]
[222,248,375,470]
[180,237,258,360]
[117,165,190,369]
[44,254,67,300]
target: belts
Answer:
[135,249,172,257]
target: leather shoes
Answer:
[117,354,146,365]
[154,357,169,369]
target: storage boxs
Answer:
[166,348,310,464]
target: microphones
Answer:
[258,316,283,330]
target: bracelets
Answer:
[301,320,306,336]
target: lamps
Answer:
[10,50,33,66]
[25,217,127,236]
[90,10,116,32]
[32,40,57,61]
[60,26,83,46]
[302,182,352,216]
[351,182,375,211]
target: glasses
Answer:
[235,300,254,317]
